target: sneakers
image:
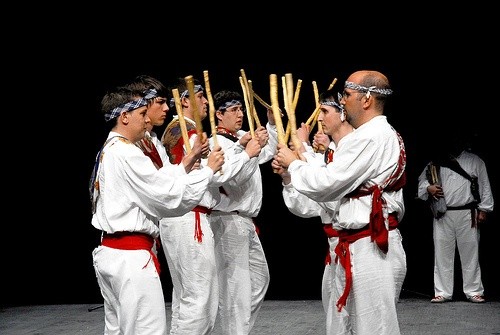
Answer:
[468,296,484,302]
[430,296,448,303]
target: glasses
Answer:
[221,107,245,114]
[343,91,366,99]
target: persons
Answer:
[418,150,494,302]
[158,78,261,335]
[88,88,209,335]
[271,70,407,335]
[206,89,285,335]
[126,75,224,248]
